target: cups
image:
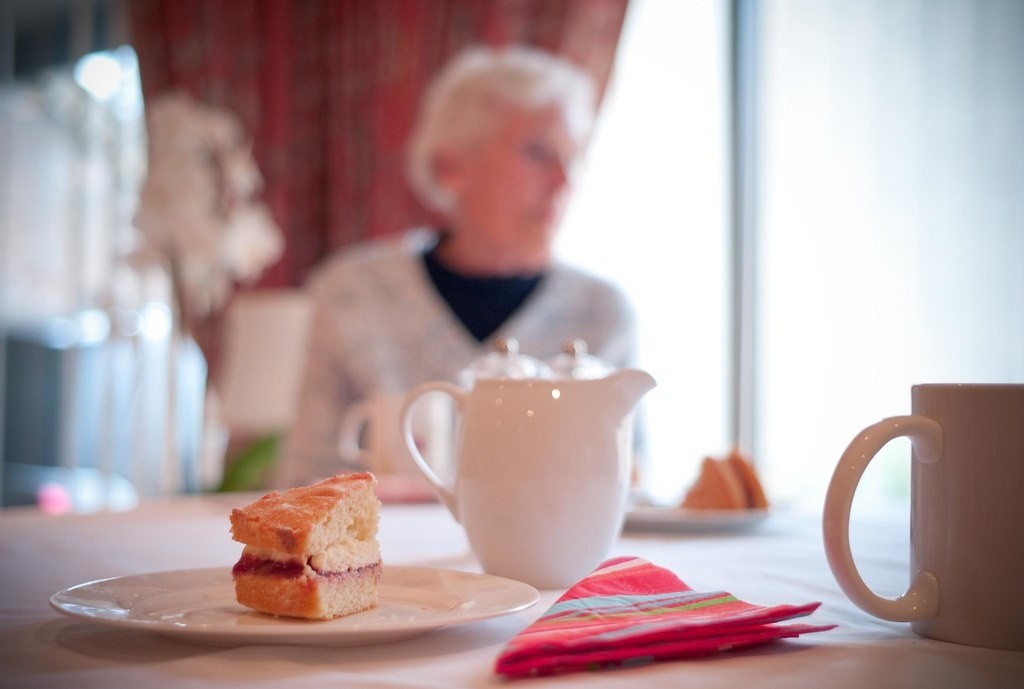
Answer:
[401,368,655,589]
[822,382,1023,648]
[338,389,449,502]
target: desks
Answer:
[0,492,1024,689]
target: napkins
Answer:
[494,559,842,677]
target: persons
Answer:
[285,44,654,504]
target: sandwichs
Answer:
[228,471,385,621]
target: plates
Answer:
[619,500,790,534]
[47,568,542,646]
